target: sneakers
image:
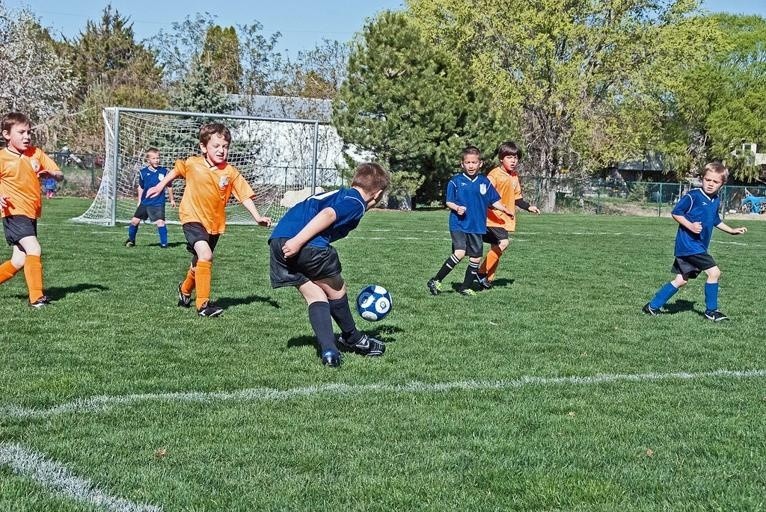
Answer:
[195,306,224,316]
[321,350,341,367]
[427,278,441,296]
[121,240,136,247]
[459,273,492,296]
[28,296,52,308]
[704,310,731,322]
[177,282,192,308]
[339,333,386,357]
[642,302,662,316]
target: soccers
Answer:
[354,285,393,323]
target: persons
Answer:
[266,161,391,367]
[0,111,62,308]
[144,122,272,317]
[640,162,748,322]
[123,148,177,247]
[476,143,540,284]
[44,177,56,197]
[428,145,513,298]
